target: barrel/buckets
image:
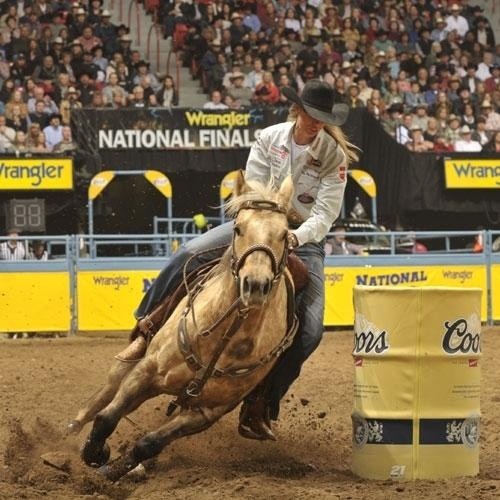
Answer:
[350,285,484,484]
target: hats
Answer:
[282,80,349,125]
[115,24,133,42]
[72,1,111,17]
[445,113,474,135]
[325,4,339,14]
[448,60,499,108]
[47,113,63,122]
[407,124,423,138]
[387,103,404,114]
[6,227,46,247]
[62,87,81,97]
[451,3,489,24]
[418,17,448,36]
[51,37,103,54]
[332,217,351,231]
[134,60,150,68]
[228,71,244,83]
[255,86,271,96]
[341,51,391,72]
[230,3,256,20]
[278,28,320,49]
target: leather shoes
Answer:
[114,336,147,362]
[239,401,276,440]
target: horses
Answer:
[65,169,299,482]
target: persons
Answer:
[144,1,499,152]
[326,224,363,256]
[113,77,365,442]
[0,0,178,154]
[405,230,426,252]
[466,225,486,252]
[0,230,60,339]
